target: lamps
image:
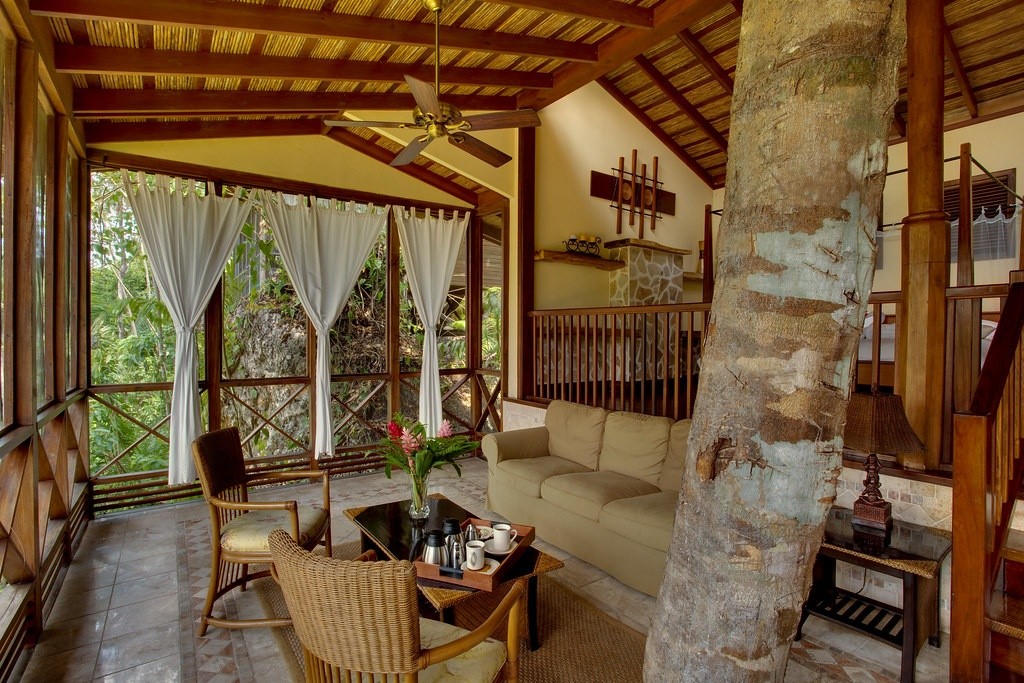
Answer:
[843,392,925,533]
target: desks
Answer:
[794,505,951,683]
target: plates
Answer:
[483,539,518,555]
[461,558,500,575]
[476,525,493,539]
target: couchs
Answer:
[482,400,692,601]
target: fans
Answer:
[322,0,541,167]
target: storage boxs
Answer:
[412,518,536,592]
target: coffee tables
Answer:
[342,493,565,653]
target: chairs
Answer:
[269,535,525,683]
[189,425,330,638]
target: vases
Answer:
[408,471,431,519]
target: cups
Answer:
[493,524,517,551]
[466,540,485,570]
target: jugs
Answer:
[409,530,449,567]
[443,518,465,569]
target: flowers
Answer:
[359,413,481,478]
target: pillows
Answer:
[661,416,695,493]
[598,410,670,496]
[545,400,608,471]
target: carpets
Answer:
[253,541,646,683]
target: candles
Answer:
[570,234,576,239]
[588,234,595,242]
[580,233,586,241]
